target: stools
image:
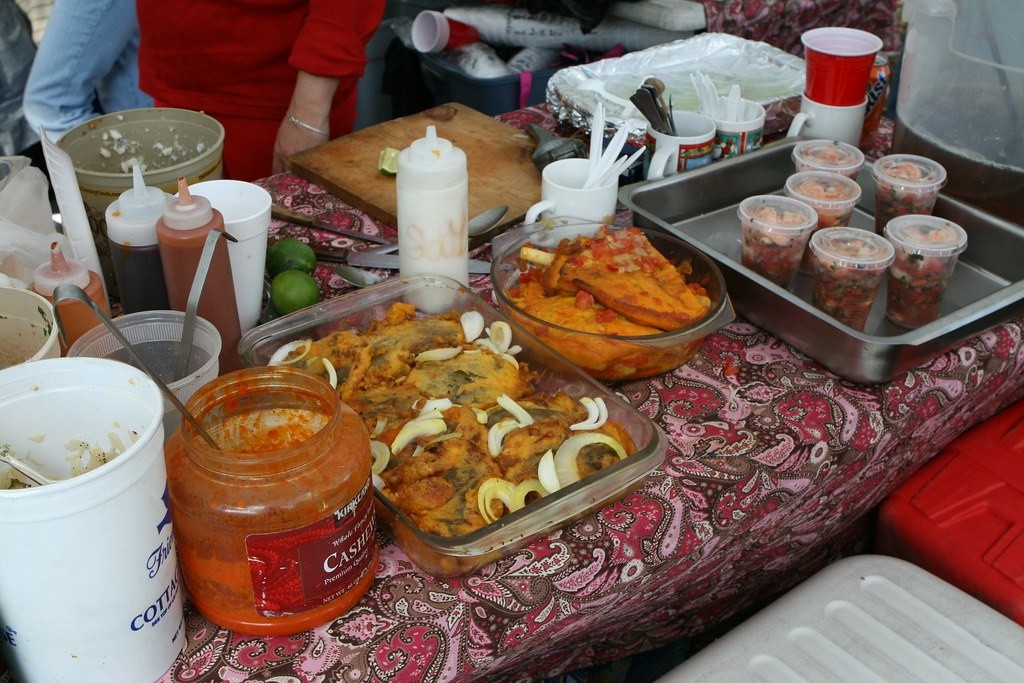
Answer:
[656,554,1024,683]
[868,400,1024,626]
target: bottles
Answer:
[105,164,177,316]
[26,242,110,357]
[397,126,469,313]
[156,176,243,377]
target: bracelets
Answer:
[289,116,329,136]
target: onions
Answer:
[267,309,628,523]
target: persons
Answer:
[24,0,154,142]
[0,0,58,215]
[135,0,387,182]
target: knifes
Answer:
[306,243,511,274]
[272,203,390,245]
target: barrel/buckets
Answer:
[890,0,1024,228]
[0,357,184,683]
[55,108,225,302]
[0,286,61,372]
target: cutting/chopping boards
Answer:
[289,101,542,238]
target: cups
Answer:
[737,194,819,292]
[166,366,379,638]
[783,140,865,276]
[809,227,895,333]
[787,27,883,149]
[697,98,766,162]
[173,179,272,336]
[411,7,694,79]
[66,310,222,446]
[644,111,717,181]
[525,158,619,248]
[871,154,969,329]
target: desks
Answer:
[166,95,1024,683]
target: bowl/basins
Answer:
[490,216,736,383]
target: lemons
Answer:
[379,147,401,173]
[269,238,316,273]
[271,270,318,315]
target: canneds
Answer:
[860,56,890,150]
[165,367,380,637]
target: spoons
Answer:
[367,205,509,253]
